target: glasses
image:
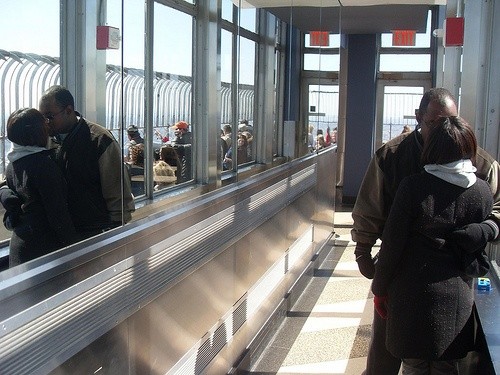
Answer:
[41,108,65,119]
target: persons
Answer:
[125,122,194,197]
[308,125,337,152]
[6,108,80,269]
[371,117,493,375]
[351,88,500,375]
[0,86,135,238]
[220,120,256,171]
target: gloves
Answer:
[0,187,20,214]
[356,254,375,278]
[449,223,494,253]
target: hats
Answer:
[171,121,188,129]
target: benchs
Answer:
[151,142,191,184]
[132,175,178,181]
